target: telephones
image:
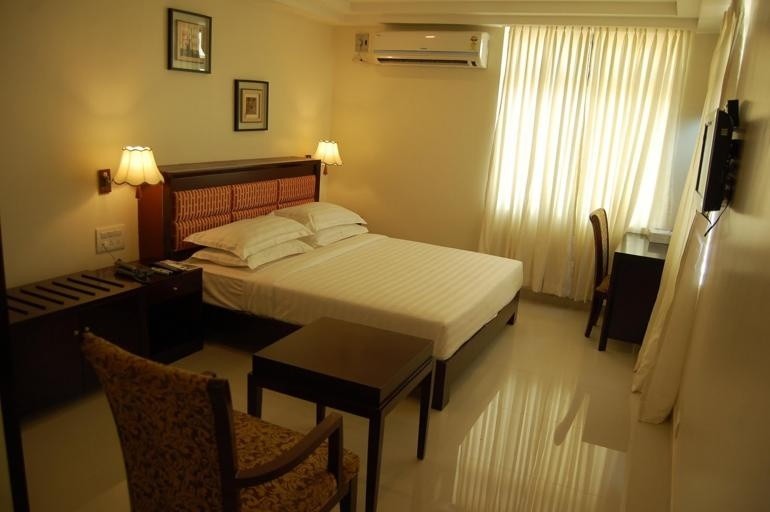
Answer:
[116,262,153,279]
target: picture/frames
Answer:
[167,7,212,73]
[235,79,268,131]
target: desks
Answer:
[599,232,670,352]
[247,318,437,512]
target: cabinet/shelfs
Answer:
[6,259,141,420]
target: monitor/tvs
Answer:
[692,108,734,214]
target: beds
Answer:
[138,156,523,411]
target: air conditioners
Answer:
[372,31,490,69]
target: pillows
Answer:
[182,198,366,270]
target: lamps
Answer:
[314,140,342,174]
[112,146,164,198]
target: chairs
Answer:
[585,209,610,337]
[75,329,358,512]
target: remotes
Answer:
[152,266,173,276]
[148,258,183,273]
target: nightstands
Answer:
[97,259,203,367]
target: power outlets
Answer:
[97,170,111,194]
[356,33,369,51]
